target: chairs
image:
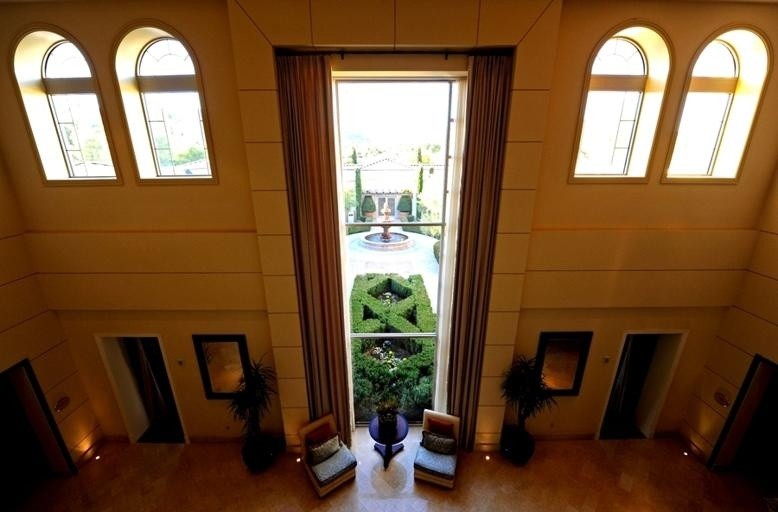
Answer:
[413,408,461,488]
[298,414,357,498]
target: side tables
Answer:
[369,413,408,468]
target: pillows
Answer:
[426,416,455,438]
[306,431,342,465]
[419,430,457,454]
[305,422,335,461]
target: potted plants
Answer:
[225,351,279,474]
[498,350,559,465]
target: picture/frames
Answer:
[534,331,593,397]
[191,333,250,400]
